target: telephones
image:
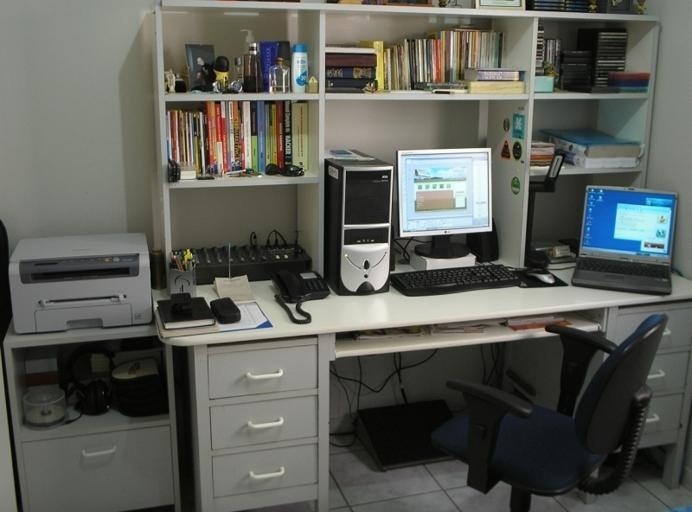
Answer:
[270,270,330,303]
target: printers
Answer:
[8,232,152,334]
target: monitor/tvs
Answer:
[397,148,493,260]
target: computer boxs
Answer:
[325,149,394,296]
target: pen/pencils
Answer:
[170,248,198,272]
[432,89,469,94]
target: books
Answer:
[165,100,310,178]
[535,241,565,251]
[155,296,217,330]
[326,24,527,96]
[544,252,574,264]
[531,125,645,171]
[350,314,574,341]
[532,1,608,14]
[329,149,353,158]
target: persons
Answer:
[195,54,217,87]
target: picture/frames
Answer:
[473,0,528,12]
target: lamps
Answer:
[525,150,569,272]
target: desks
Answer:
[145,238,691,509]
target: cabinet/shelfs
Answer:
[323,5,538,284]
[484,14,660,273]
[5,316,182,509]
[154,2,323,296]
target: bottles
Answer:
[150,248,167,289]
[268,56,290,93]
[234,56,243,82]
[243,42,264,93]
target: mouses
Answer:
[522,268,555,286]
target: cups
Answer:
[259,40,279,91]
[169,266,196,298]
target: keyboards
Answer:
[388,264,519,297]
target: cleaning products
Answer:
[245,44,261,92]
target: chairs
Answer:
[429,308,669,510]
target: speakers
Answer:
[466,218,499,263]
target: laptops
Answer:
[571,185,678,295]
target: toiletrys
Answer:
[240,29,255,53]
[292,44,309,93]
